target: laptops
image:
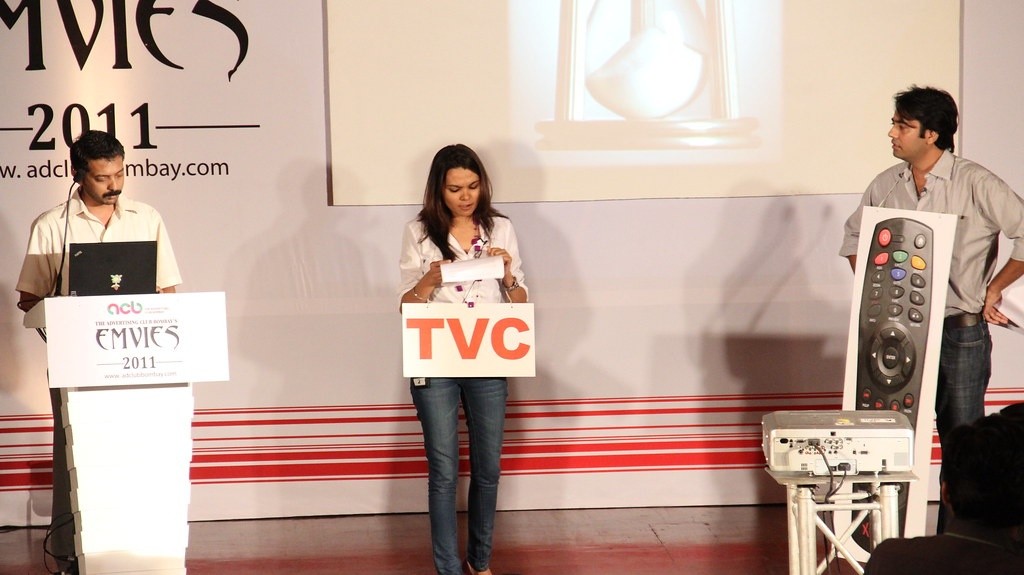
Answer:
[69,241,158,296]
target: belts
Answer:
[943,313,982,329]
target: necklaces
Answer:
[454,217,483,308]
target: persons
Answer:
[15,131,182,575]
[838,86,1024,535]
[862,402,1024,575]
[398,143,530,575]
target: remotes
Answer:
[851,218,934,552]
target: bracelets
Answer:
[412,287,427,301]
[503,276,520,292]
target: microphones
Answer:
[56,171,82,296]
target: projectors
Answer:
[761,409,915,476]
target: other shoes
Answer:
[462,558,473,575]
